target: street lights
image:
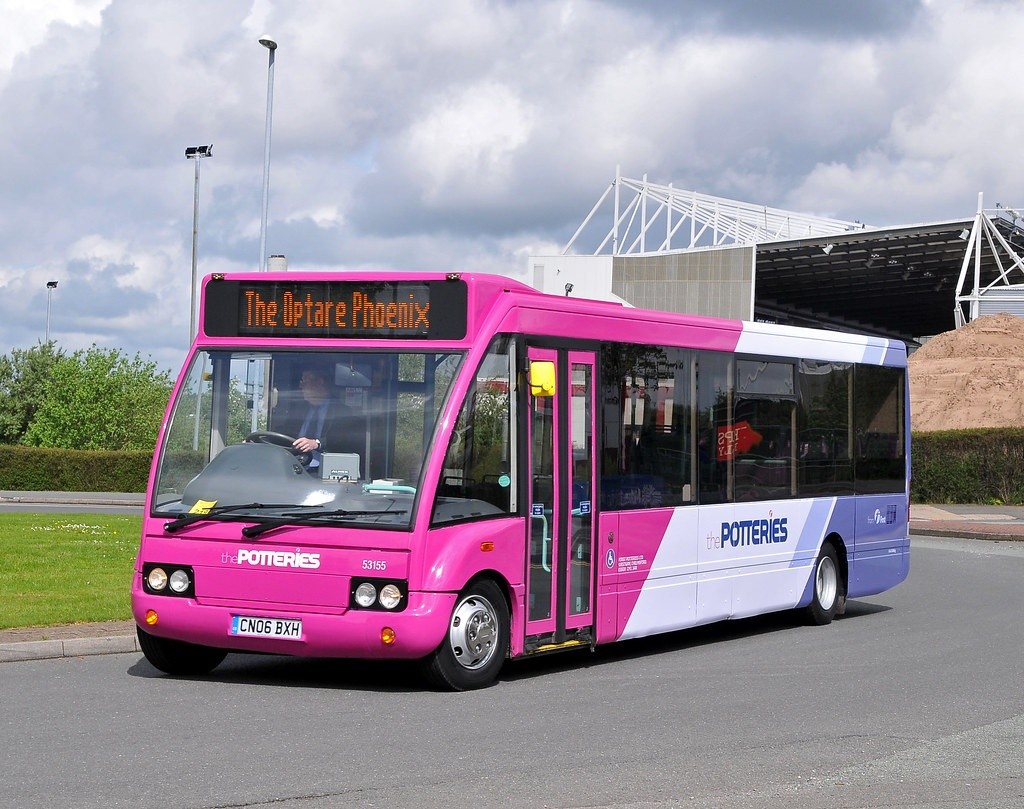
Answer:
[47,281,57,350]
[259,35,278,273]
[185,144,214,350]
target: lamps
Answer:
[900,269,911,281]
[822,238,833,255]
[864,254,874,269]
[959,228,968,241]
[933,282,943,292]
[996,245,1003,255]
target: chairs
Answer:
[442,477,479,499]
[483,475,510,510]
[533,478,552,507]
[864,433,897,462]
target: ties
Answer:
[304,405,319,441]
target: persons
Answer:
[633,433,688,507]
[273,365,352,472]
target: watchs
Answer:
[313,439,321,451]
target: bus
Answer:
[131,270,910,692]
